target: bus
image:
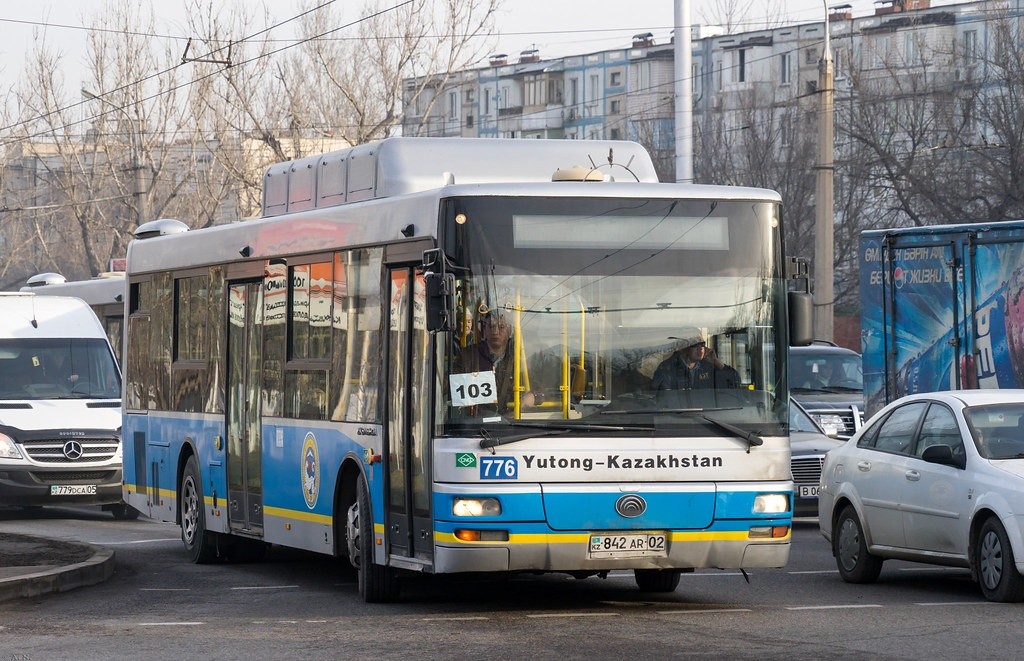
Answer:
[18,273,128,380]
[121,136,793,608]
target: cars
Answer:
[717,335,866,519]
[817,388,1024,604]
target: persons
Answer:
[31,342,75,384]
[450,307,544,412]
[803,359,841,388]
[652,326,742,390]
[298,379,332,420]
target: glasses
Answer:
[690,342,706,347]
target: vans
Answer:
[0,291,142,522]
